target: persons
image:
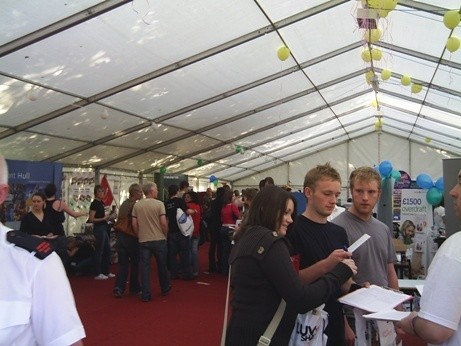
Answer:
[114,181,169,301]
[223,183,357,346]
[286,165,352,346]
[204,178,308,271]
[20,193,55,236]
[394,169,461,346]
[0,151,86,346]
[89,186,116,280]
[329,167,404,346]
[45,183,87,258]
[165,181,200,279]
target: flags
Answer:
[101,177,114,207]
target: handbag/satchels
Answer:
[176,208,194,237]
[288,303,329,346]
[113,200,138,238]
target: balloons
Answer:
[210,176,218,186]
[376,160,401,181]
[416,174,443,206]
[278,47,290,61]
[362,0,461,144]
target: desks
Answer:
[399,280,426,312]
[393,239,415,278]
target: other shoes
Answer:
[114,288,123,297]
[142,296,151,301]
[163,281,173,295]
[128,289,143,295]
[107,273,116,278]
[94,273,109,280]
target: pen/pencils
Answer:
[343,245,349,252]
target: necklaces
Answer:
[412,315,418,336]
